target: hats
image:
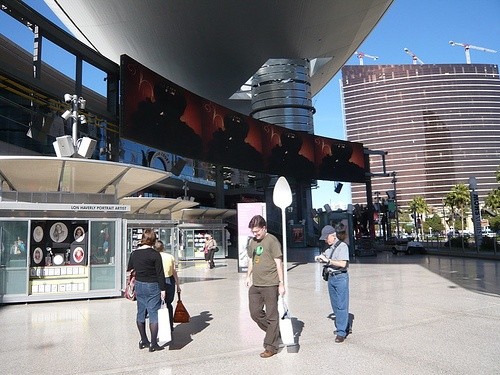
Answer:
[319,225,335,240]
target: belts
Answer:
[331,270,347,276]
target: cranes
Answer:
[404,47,425,64]
[449,39,498,64]
[353,51,379,66]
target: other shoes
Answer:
[171,327,174,331]
[260,350,276,358]
[333,330,349,342]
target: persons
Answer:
[103,235,111,262]
[154,240,181,331]
[315,225,349,343]
[245,215,286,357]
[126,228,166,352]
[10,236,24,255]
[203,234,218,269]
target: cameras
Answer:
[317,258,322,263]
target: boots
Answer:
[137,321,150,349]
[149,323,164,352]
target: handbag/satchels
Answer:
[278,294,294,344]
[145,298,172,347]
[322,265,329,281]
[214,247,219,252]
[203,247,210,261]
[173,292,191,323]
[124,268,136,301]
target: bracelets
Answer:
[329,259,332,266]
[279,281,284,283]
